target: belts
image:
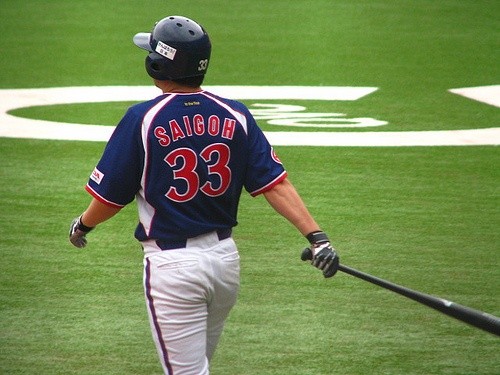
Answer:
[156,229,232,250]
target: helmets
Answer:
[133,16,212,79]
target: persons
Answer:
[67,14,340,375]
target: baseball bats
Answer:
[300,244,500,336]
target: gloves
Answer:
[305,231,339,278]
[69,215,96,248]
